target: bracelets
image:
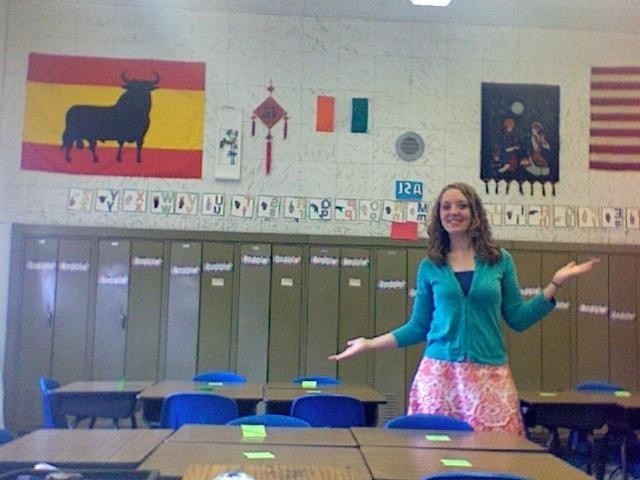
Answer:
[550,279,562,290]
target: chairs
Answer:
[194,372,250,384]
[0,430,28,478]
[388,412,472,433]
[42,378,121,429]
[571,382,623,474]
[292,394,368,427]
[224,414,313,426]
[161,392,239,425]
[421,470,526,480]
[296,376,345,386]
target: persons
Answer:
[325,185,601,440]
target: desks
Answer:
[5,427,174,471]
[263,379,379,389]
[265,390,389,429]
[135,423,373,480]
[135,382,266,427]
[48,380,156,433]
[350,429,595,479]
[517,386,639,479]
